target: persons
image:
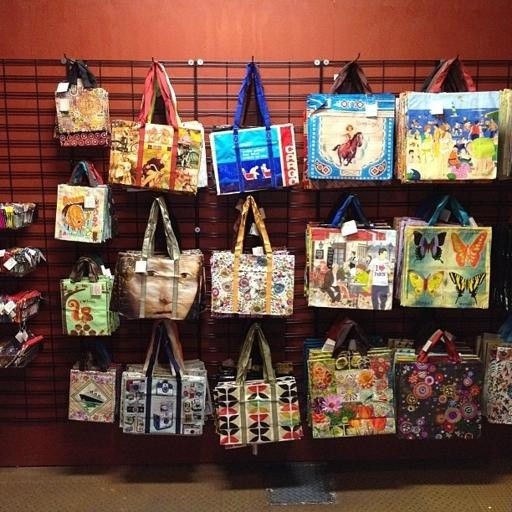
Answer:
[410,115,499,174]
[323,264,343,301]
[347,251,358,274]
[342,124,354,145]
[120,255,199,318]
[368,248,392,309]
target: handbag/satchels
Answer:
[478,317,512,427]
[65,342,117,424]
[51,163,110,245]
[62,259,115,340]
[395,59,502,188]
[397,193,494,309]
[107,60,207,196]
[303,63,397,191]
[213,324,303,448]
[303,320,397,438]
[117,321,210,438]
[210,199,297,317]
[108,200,207,321]
[393,328,485,445]
[304,197,398,312]
[51,57,111,149]
[210,64,301,196]
